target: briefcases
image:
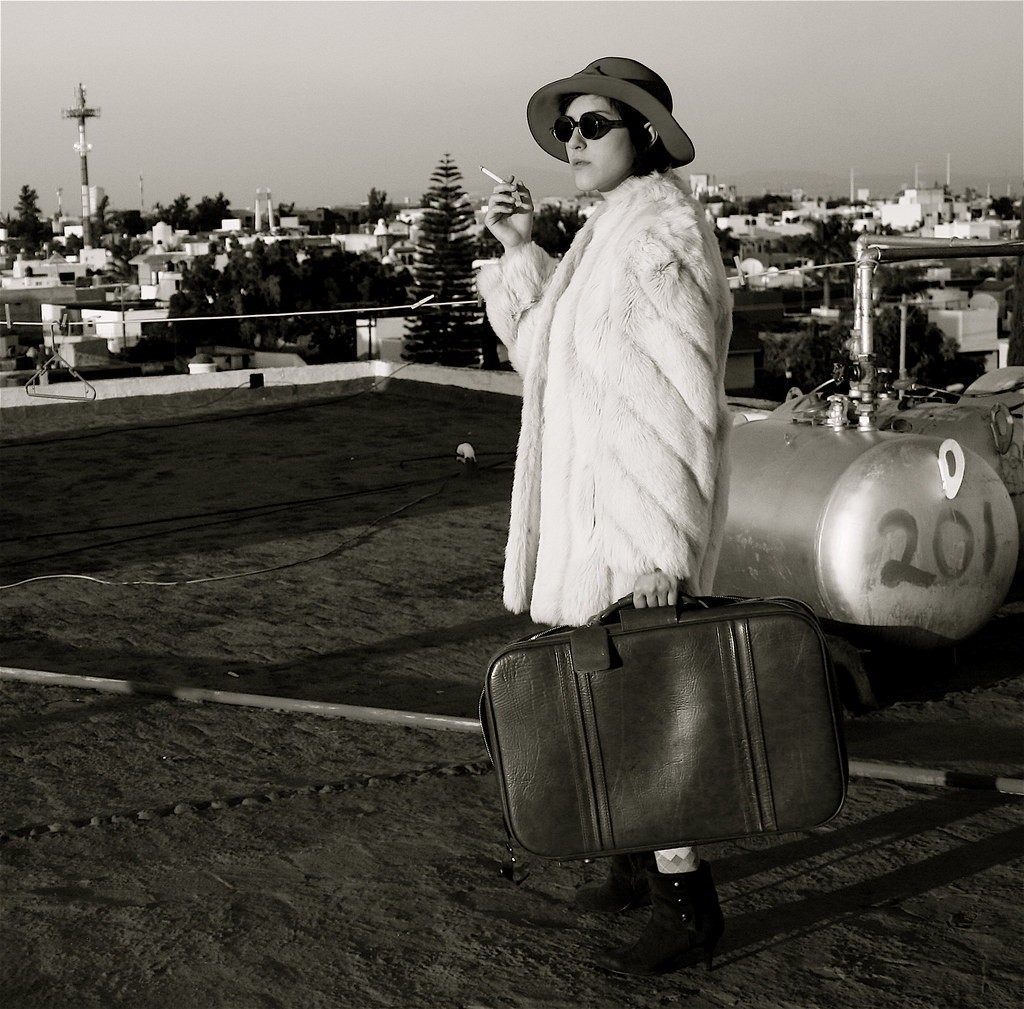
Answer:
[475,591,848,864]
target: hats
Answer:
[526,57,695,172]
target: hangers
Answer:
[25,320,96,402]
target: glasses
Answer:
[550,113,629,143]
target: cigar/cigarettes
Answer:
[479,165,516,192]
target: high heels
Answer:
[574,858,649,913]
[593,859,722,974]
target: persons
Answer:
[478,57,732,977]
